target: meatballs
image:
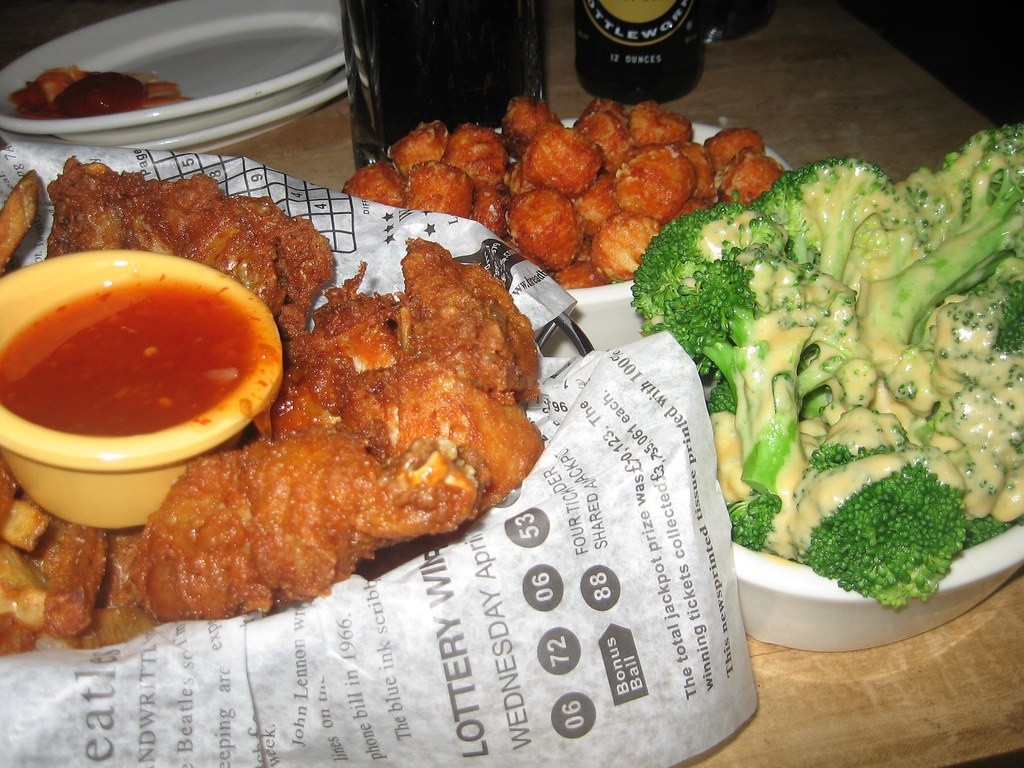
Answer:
[346,96,780,282]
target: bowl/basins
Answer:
[0,245,286,529]
[708,518,1023,654]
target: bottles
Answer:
[341,0,548,175]
[574,0,705,104]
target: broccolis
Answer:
[630,122,1024,608]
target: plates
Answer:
[439,114,796,359]
[0,66,359,153]
[0,0,352,147]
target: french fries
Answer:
[0,171,153,658]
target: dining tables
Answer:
[0,0,1024,768]
